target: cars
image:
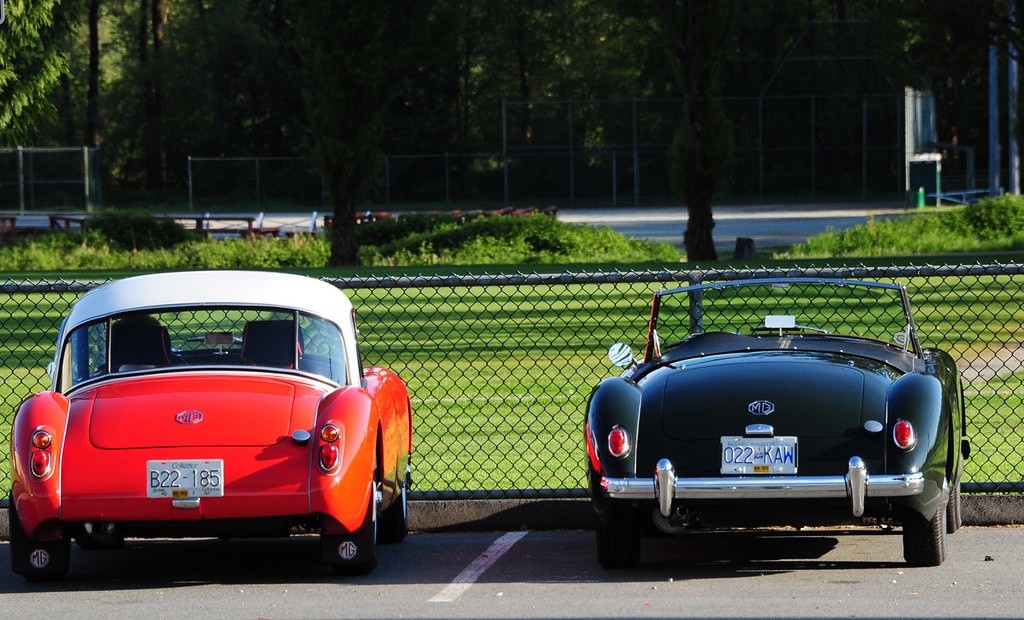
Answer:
[7,270,412,579]
[583,278,971,568]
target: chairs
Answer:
[242,319,304,371]
[103,312,175,376]
